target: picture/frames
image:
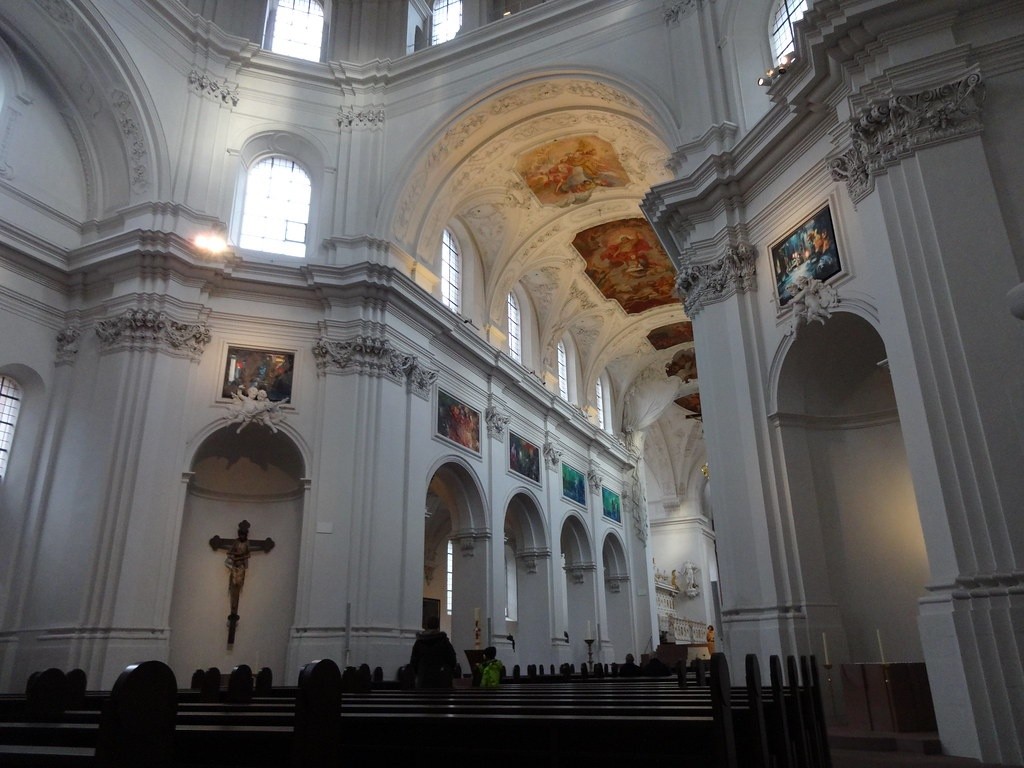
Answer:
[561,459,589,510]
[601,483,623,527]
[508,427,543,488]
[435,384,483,458]
[216,343,300,408]
[764,195,848,318]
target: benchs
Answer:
[0,654,831,768]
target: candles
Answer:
[876,629,886,664]
[822,632,830,665]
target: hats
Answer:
[483,647,496,655]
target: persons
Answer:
[409,616,462,694]
[226,385,278,433]
[619,654,672,679]
[224,527,251,613]
[678,560,697,587]
[706,625,717,658]
[785,276,840,338]
[475,647,508,692]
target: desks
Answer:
[840,662,939,732]
